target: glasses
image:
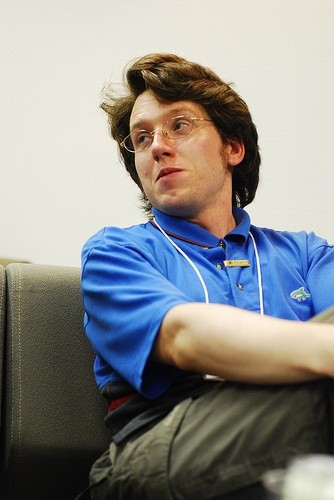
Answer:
[120,114,214,153]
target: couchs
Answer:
[0,262,114,500]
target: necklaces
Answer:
[153,218,264,315]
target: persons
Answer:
[81,54,334,500]
